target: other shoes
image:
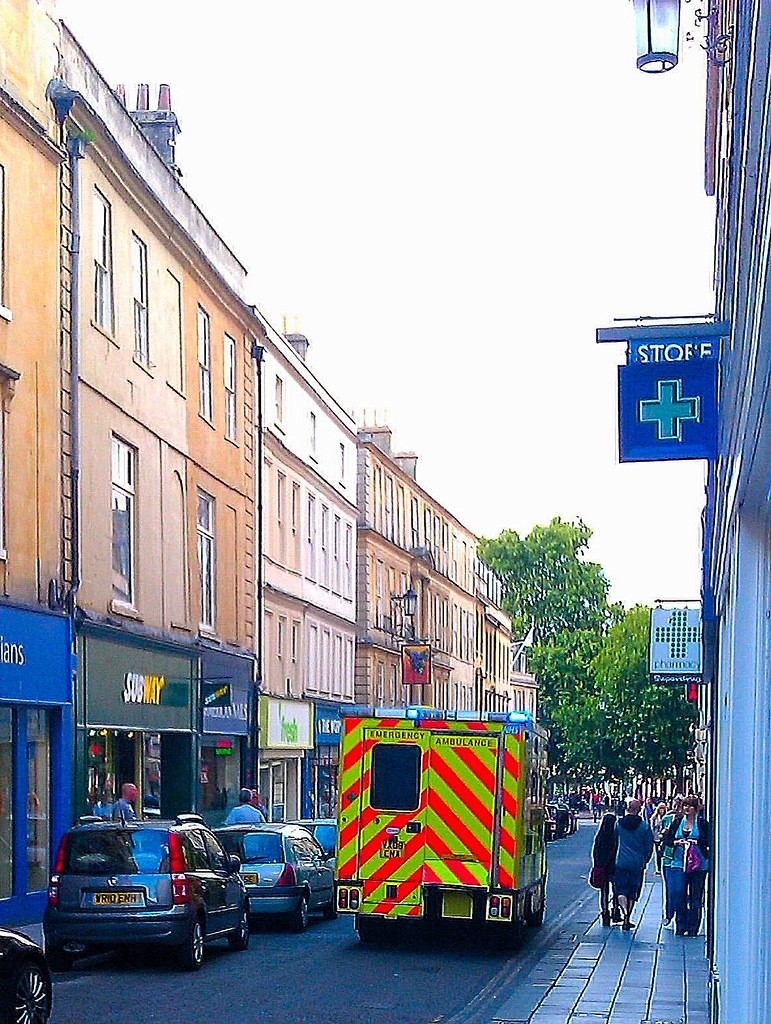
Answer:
[610,907,624,923]
[600,910,611,928]
[663,913,698,938]
[623,917,635,931]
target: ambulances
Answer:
[336,705,559,944]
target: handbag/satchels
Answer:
[589,867,606,890]
[686,842,701,873]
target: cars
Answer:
[207,820,336,930]
[545,799,578,842]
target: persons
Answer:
[614,800,654,930]
[667,796,709,935]
[21,794,46,855]
[224,789,266,824]
[307,783,338,818]
[649,802,666,874]
[247,789,269,819]
[113,783,138,819]
[554,785,704,823]
[214,786,233,808]
[661,794,684,926]
[588,814,623,925]
[86,785,116,819]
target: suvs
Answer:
[46,816,249,972]
